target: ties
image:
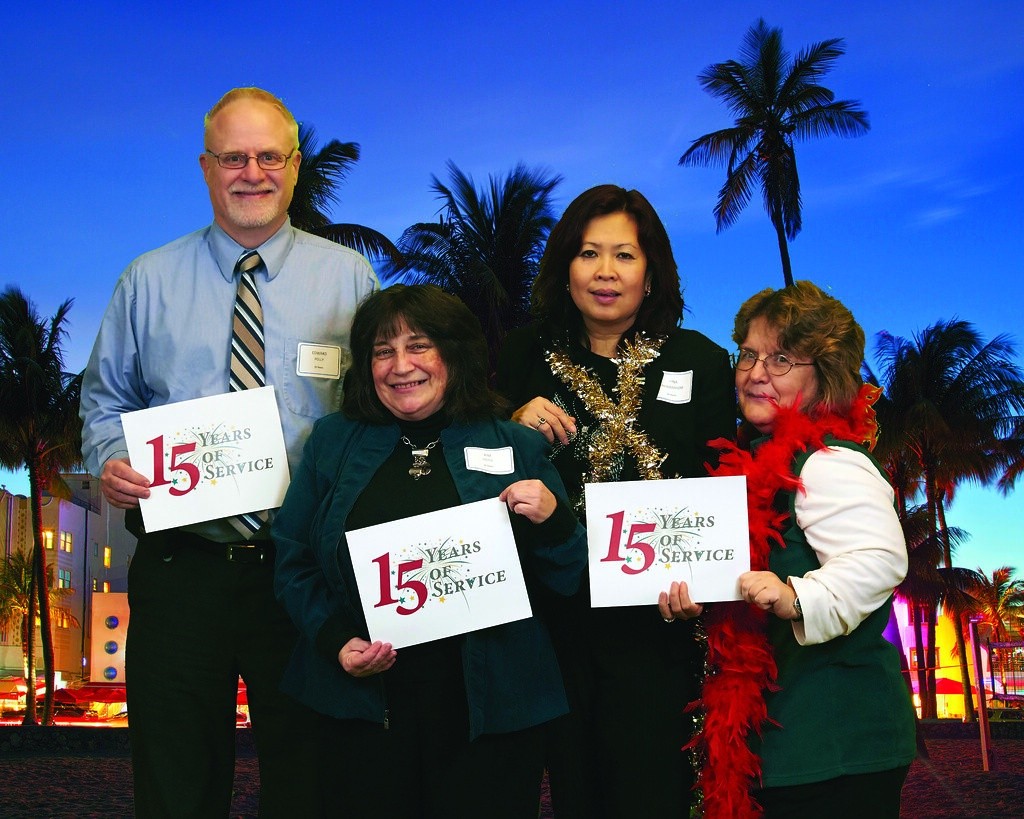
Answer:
[224,249,268,542]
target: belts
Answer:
[166,528,276,566]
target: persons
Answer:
[498,186,745,819]
[264,287,587,819]
[79,86,384,819]
[658,282,920,819]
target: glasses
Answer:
[206,148,294,170]
[732,347,818,376]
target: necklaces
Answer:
[400,436,441,480]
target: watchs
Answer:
[793,596,803,622]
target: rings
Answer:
[663,616,676,623]
[536,418,547,430]
